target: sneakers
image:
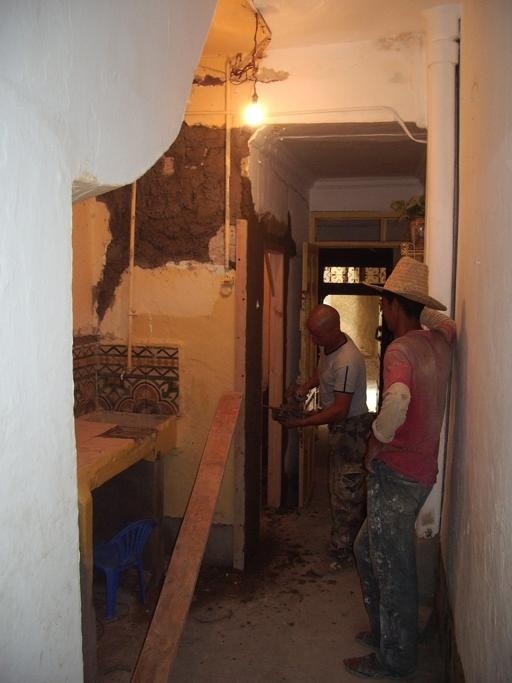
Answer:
[342,623,406,679]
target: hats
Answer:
[361,256,448,311]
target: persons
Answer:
[276,302,371,576]
[341,257,456,683]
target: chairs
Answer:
[89,518,156,621]
